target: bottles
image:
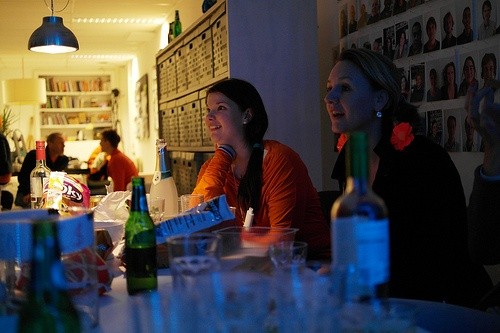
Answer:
[30,140,53,209]
[123,177,157,296]
[14,216,82,333]
[331,131,393,306]
[149,139,177,224]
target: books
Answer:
[37,75,115,123]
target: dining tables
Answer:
[0,212,500,333]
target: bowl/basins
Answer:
[93,222,123,244]
[214,226,298,261]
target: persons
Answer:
[0,133,14,184]
[98,130,138,190]
[14,132,69,209]
[334,1,499,151]
[188,75,327,263]
[324,42,468,304]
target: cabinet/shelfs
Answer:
[34,71,117,153]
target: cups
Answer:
[110,257,414,333]
[166,232,222,280]
[269,242,307,274]
[179,194,205,214]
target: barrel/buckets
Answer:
[0,208,98,333]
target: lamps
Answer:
[27,0,78,55]
